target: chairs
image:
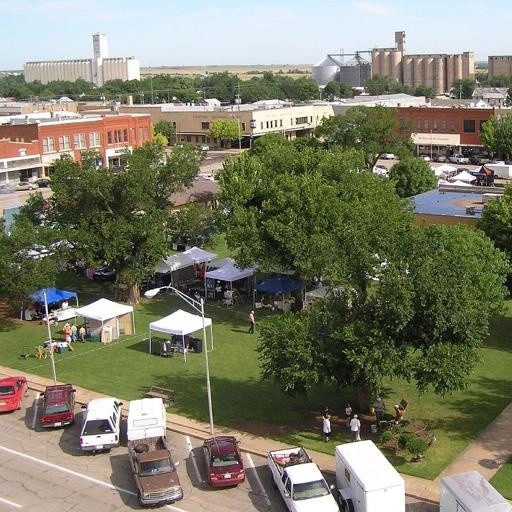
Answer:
[223,297,233,308]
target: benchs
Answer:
[145,385,175,407]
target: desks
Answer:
[55,308,80,321]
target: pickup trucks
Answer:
[268,447,340,512]
[127,436,183,509]
[449,153,469,165]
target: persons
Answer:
[61,300,69,310]
[40,303,47,320]
[322,406,329,419]
[350,414,361,441]
[393,404,403,425]
[248,310,256,334]
[215,281,223,303]
[323,413,332,442]
[345,403,353,427]
[62,323,86,343]
[373,398,385,426]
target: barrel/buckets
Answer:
[369,404,375,414]
[371,425,377,433]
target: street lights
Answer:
[143,285,214,436]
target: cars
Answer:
[0,377,123,453]
[15,177,52,191]
[202,436,245,488]
[176,143,210,151]
[375,153,446,174]
